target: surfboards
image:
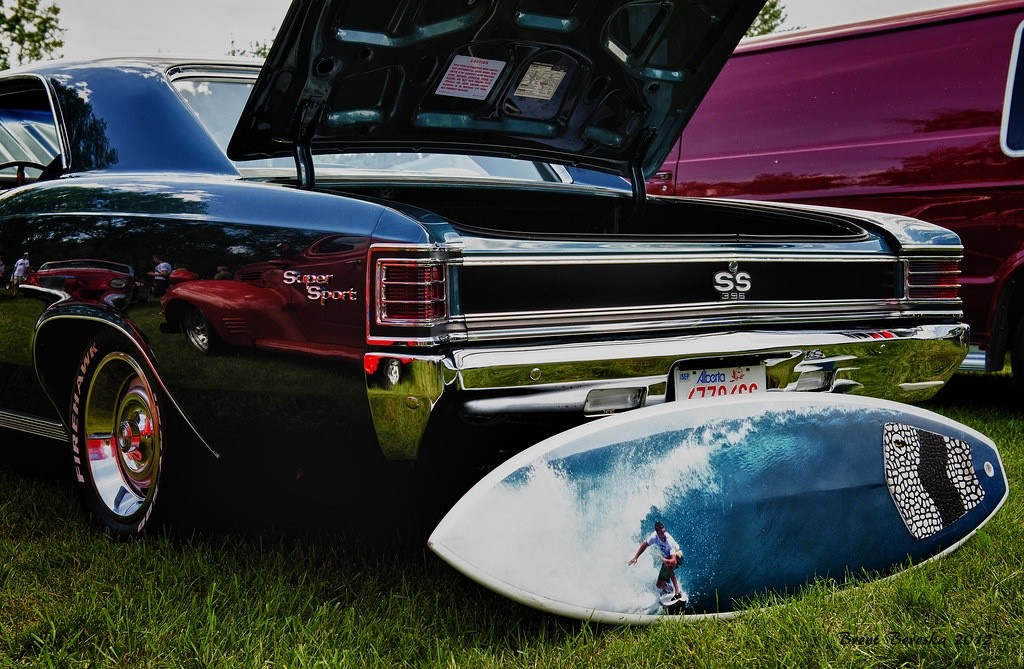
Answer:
[660,590,688,611]
[425,390,1010,629]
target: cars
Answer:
[0,0,976,537]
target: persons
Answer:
[628,522,685,601]
[214,267,234,281]
[0,252,30,296]
[147,256,172,315]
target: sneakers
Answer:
[660,590,671,596]
[671,593,682,601]
